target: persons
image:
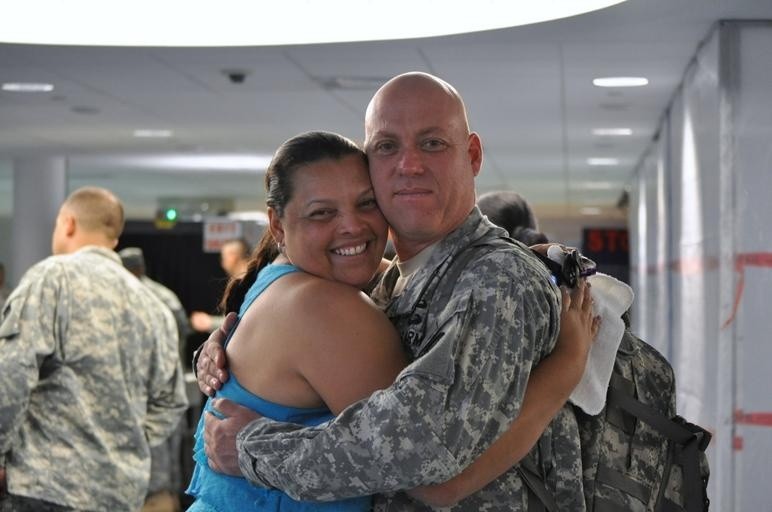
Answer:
[119,246,190,512]
[190,236,255,335]
[0,185,190,512]
[189,70,590,512]
[474,191,553,251]
[181,129,606,512]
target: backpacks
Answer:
[434,234,712,512]
